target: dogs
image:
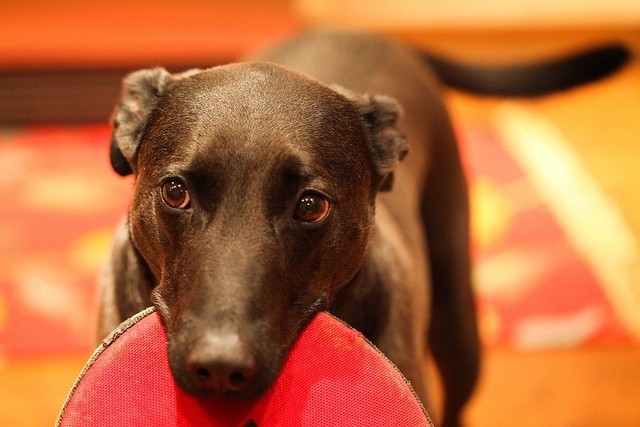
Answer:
[95,26,635,427]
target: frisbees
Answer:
[54,306,436,427]
